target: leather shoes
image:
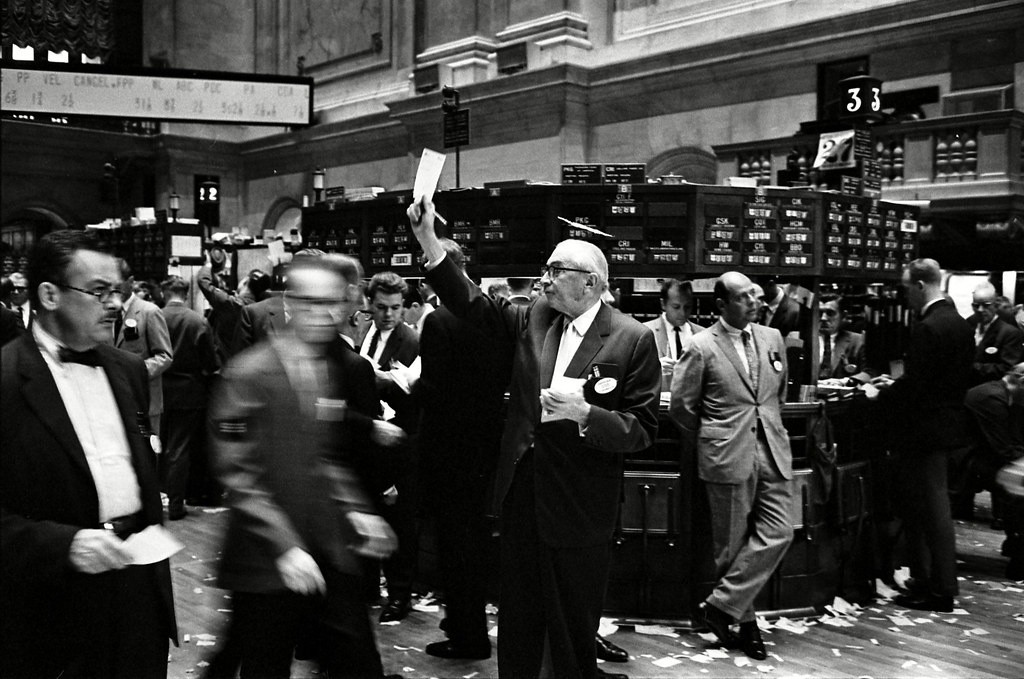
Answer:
[379,598,412,622]
[595,632,628,660]
[692,602,740,650]
[739,628,766,660]
[895,590,954,612]
[598,669,628,679]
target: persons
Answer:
[201,256,398,679]
[0,223,182,679]
[343,241,552,660]
[407,195,664,679]
[112,250,288,521]
[641,280,708,393]
[667,272,797,660]
[881,258,969,613]
[964,281,1024,581]
[786,291,874,390]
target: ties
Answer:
[18,307,23,323]
[742,330,760,395]
[821,335,831,369]
[113,310,123,344]
[550,322,574,387]
[367,329,380,359]
[674,326,682,360]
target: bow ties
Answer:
[58,344,104,367]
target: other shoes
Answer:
[169,509,188,520]
[425,640,491,659]
[904,579,924,593]
[991,518,1006,530]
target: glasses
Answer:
[11,287,28,293]
[540,265,592,281]
[55,284,126,304]
[350,310,373,321]
[972,302,995,308]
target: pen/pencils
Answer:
[423,197,448,224]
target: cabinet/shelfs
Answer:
[86,221,204,289]
[301,180,921,386]
